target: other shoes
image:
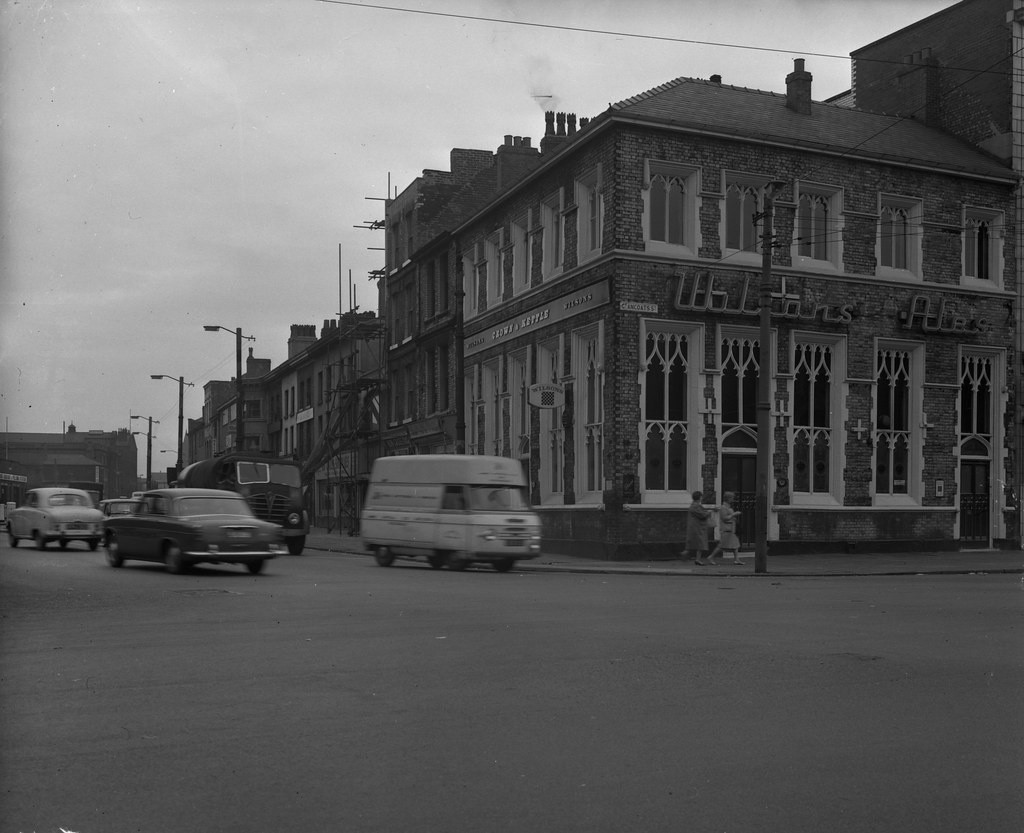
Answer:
[733,561,746,565]
[707,556,716,565]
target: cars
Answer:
[83,489,147,516]
[4,487,107,552]
[104,488,285,575]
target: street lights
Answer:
[203,323,257,450]
[151,372,195,472]
[755,178,790,576]
[129,414,160,490]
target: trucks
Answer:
[358,452,543,574]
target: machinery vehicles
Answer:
[168,449,310,553]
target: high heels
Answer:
[695,561,705,566]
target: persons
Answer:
[679,492,712,566]
[706,492,745,565]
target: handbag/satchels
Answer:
[706,512,717,529]
